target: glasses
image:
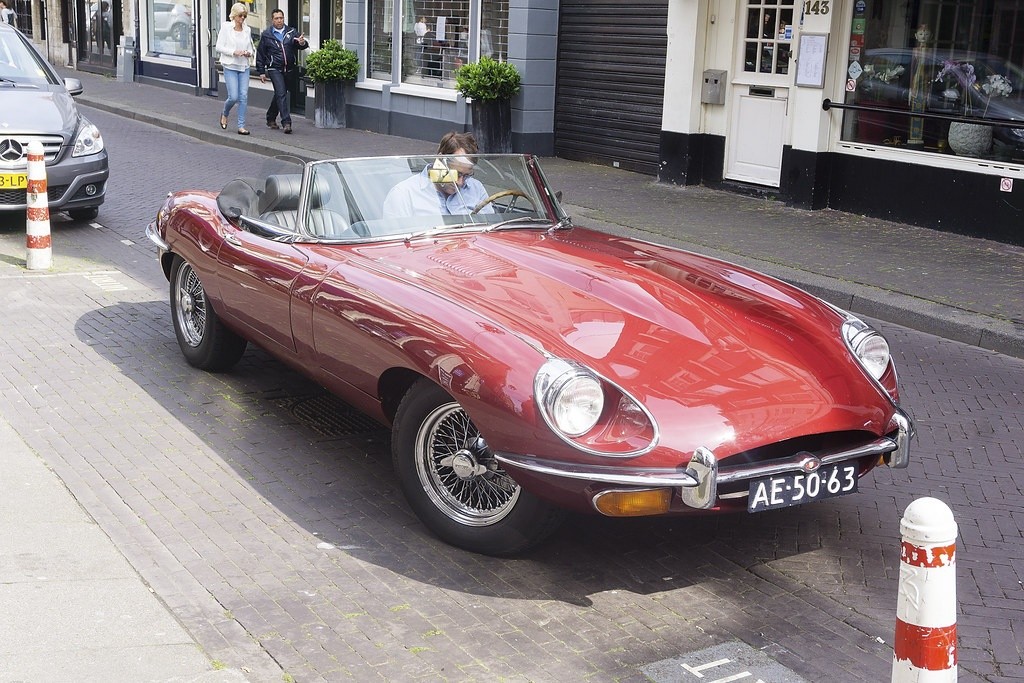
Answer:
[238,14,247,18]
[458,169,474,179]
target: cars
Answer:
[0,24,111,225]
[90,1,114,41]
[859,49,1023,161]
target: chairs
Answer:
[259,174,348,242]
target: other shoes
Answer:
[283,123,292,133]
[220,113,228,129]
[238,130,250,135]
[267,121,279,129]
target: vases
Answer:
[948,122,993,157]
[854,100,889,144]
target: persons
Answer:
[96,2,111,54]
[383,131,495,217]
[256,9,308,133]
[414,14,431,74]
[216,2,253,135]
[0,0,18,29]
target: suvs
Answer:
[154,2,192,40]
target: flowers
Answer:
[927,60,1013,120]
[864,65,904,101]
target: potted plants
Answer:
[454,57,520,161]
[304,38,362,129]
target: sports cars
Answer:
[146,148,919,559]
